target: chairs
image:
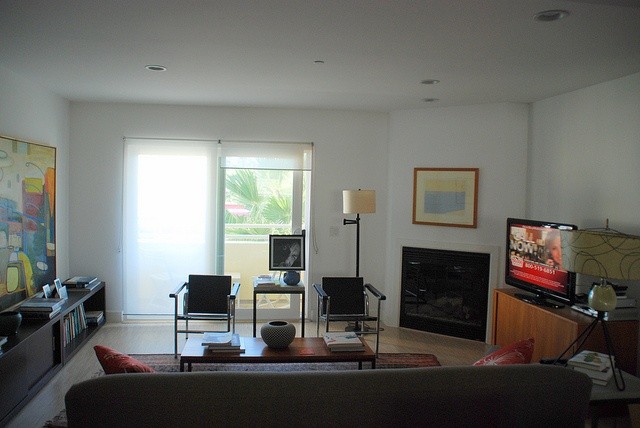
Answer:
[169,275,240,358]
[311,276,386,358]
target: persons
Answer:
[545,230,568,274]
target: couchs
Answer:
[65,365,592,428]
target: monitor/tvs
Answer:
[505,217,578,310]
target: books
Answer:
[322,332,365,353]
[567,350,616,386]
[64,303,88,347]
[592,281,636,308]
[86,310,105,326]
[19,297,66,320]
[201,331,245,354]
[254,275,281,288]
[62,275,101,291]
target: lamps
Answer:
[343,189,376,333]
[553,219,640,391]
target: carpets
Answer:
[41,353,442,428]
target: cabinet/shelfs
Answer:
[0,282,107,428]
[492,287,640,376]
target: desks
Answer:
[179,337,376,372]
[253,277,305,338]
[544,363,640,428]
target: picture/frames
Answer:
[412,168,479,228]
[58,286,68,301]
[270,234,306,271]
[1,135,56,302]
[54,278,61,298]
[43,283,51,298]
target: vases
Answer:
[283,271,300,285]
[261,320,296,350]
[0,311,23,336]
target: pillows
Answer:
[93,345,157,373]
[472,337,535,366]
